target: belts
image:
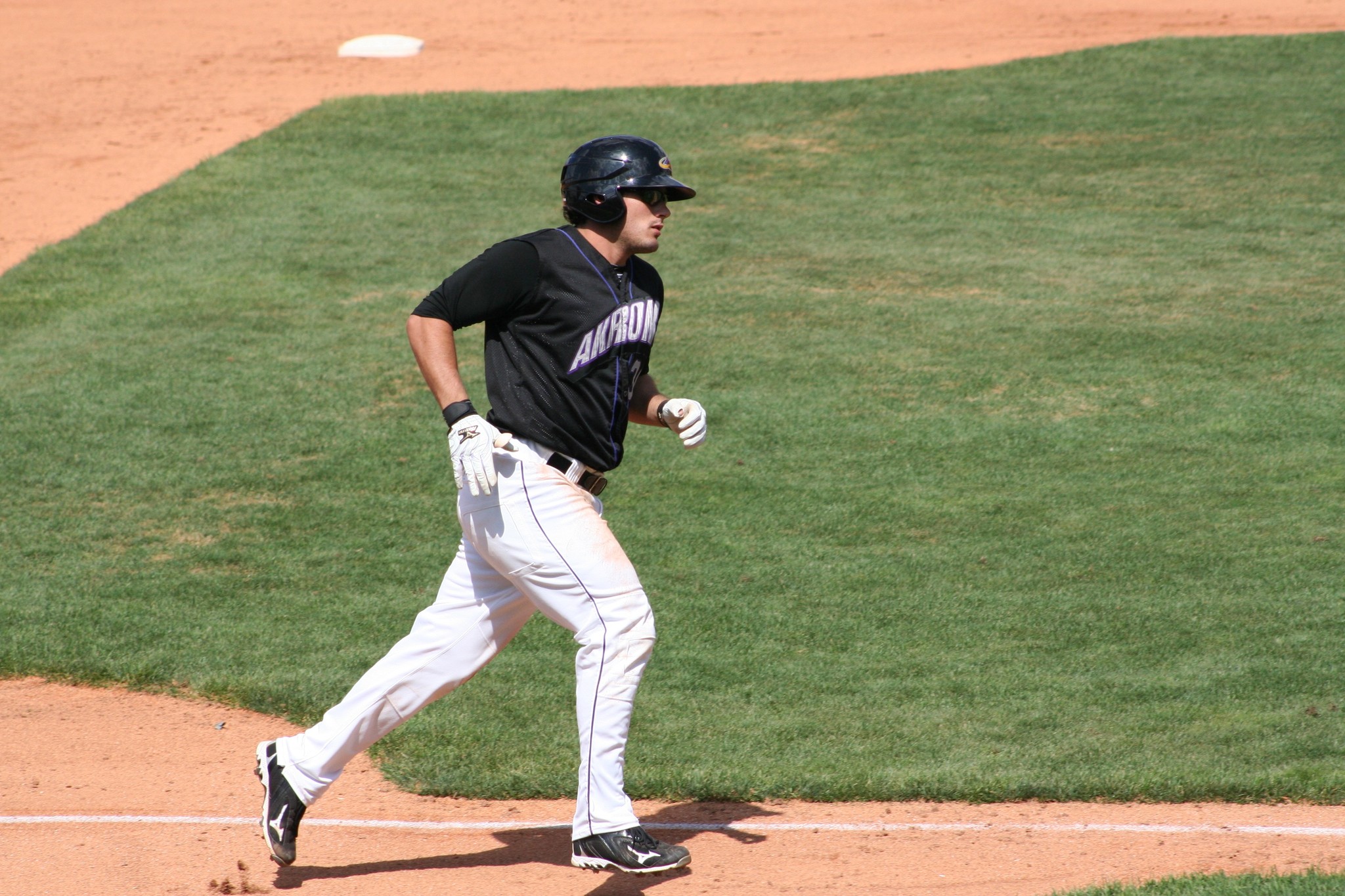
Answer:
[547,452,607,497]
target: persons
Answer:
[255,135,707,873]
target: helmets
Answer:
[561,136,696,225]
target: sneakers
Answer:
[571,826,691,873]
[256,741,307,867]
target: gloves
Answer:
[442,399,519,497]
[657,398,706,449]
